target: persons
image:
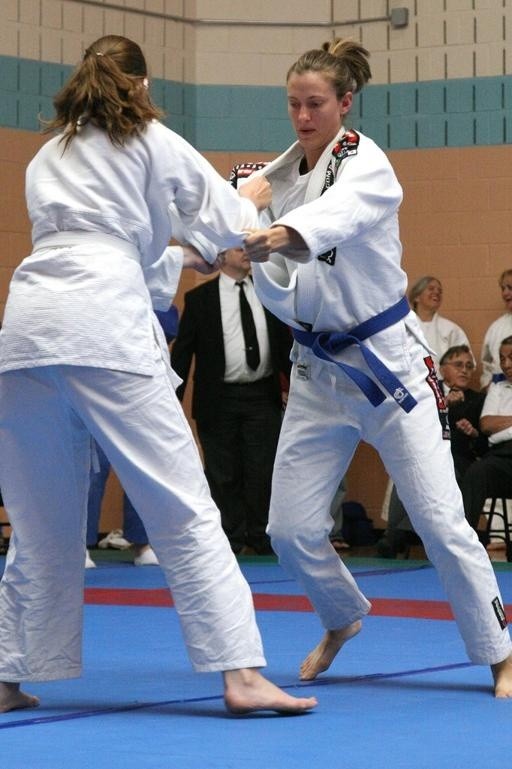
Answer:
[172,39,510,700]
[457,334,511,554]
[2,34,318,721]
[372,345,481,561]
[76,298,180,568]
[173,245,296,558]
[380,277,474,528]
[478,267,510,552]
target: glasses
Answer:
[442,360,475,370]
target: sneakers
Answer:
[132,548,162,568]
[82,548,98,570]
[95,527,132,551]
[225,534,276,556]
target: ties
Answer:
[234,278,261,375]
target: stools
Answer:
[478,492,512,564]
